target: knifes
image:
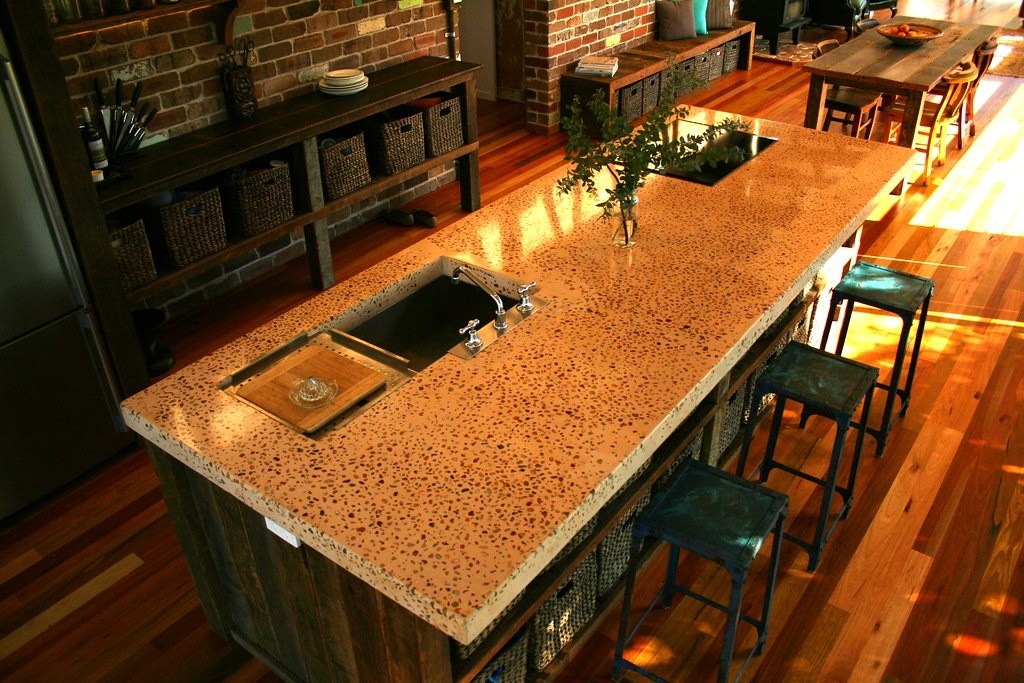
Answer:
[92,77,157,128]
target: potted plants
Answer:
[554,46,757,250]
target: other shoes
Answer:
[389,211,413,226]
[413,208,437,228]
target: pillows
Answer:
[706,0,734,31]
[655,0,697,41]
[693,0,709,36]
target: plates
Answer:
[318,69,369,97]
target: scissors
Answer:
[218,46,237,66]
[239,39,255,65]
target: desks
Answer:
[800,14,1002,150]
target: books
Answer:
[573,56,619,77]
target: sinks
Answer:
[328,255,542,372]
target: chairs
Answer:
[852,18,890,111]
[899,37,999,151]
[812,38,882,141]
[881,61,979,190]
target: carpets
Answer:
[985,27,1024,78]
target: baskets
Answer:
[372,111,425,175]
[107,219,157,294]
[456,303,811,683]
[420,91,465,157]
[160,186,227,269]
[611,40,741,123]
[230,162,294,236]
[318,128,372,201]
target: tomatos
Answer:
[889,25,917,37]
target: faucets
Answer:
[450,265,507,332]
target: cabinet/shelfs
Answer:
[561,20,756,140]
[90,55,483,316]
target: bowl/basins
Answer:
[876,22,944,47]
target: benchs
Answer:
[803,0,898,42]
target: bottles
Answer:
[79,106,112,179]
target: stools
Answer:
[730,341,881,575]
[603,456,790,683]
[796,257,936,460]
